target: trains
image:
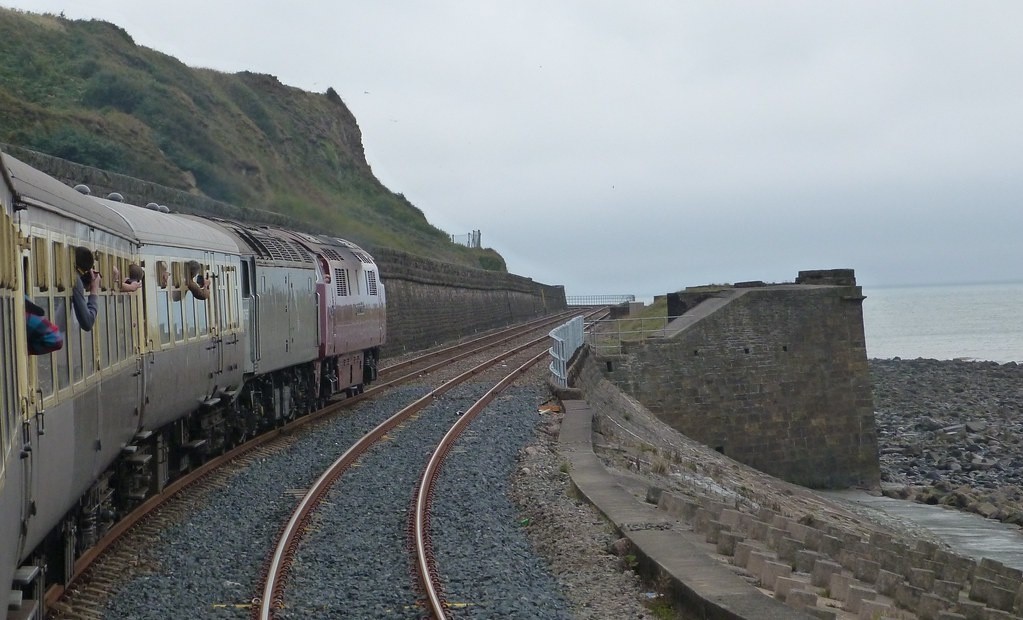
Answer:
[0,151,388,620]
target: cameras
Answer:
[81,273,92,286]
[197,276,204,285]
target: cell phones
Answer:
[126,279,139,285]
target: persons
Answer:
[73,247,100,332]
[111,266,120,295]
[188,260,211,300]
[24,295,64,356]
[161,262,170,289]
[122,264,144,292]
